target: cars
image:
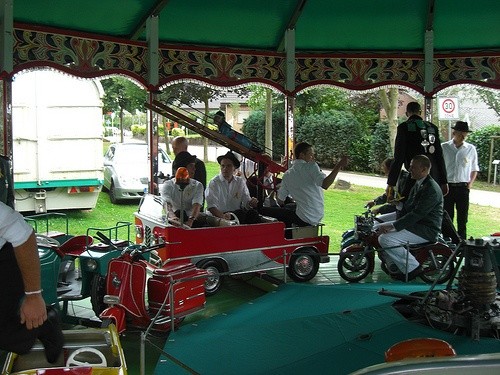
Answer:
[103,143,176,205]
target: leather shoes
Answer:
[402,265,424,282]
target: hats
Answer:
[172,150,197,169]
[450,120,473,134]
[216,151,240,169]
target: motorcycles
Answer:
[336,203,459,288]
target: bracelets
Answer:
[188,215,196,220]
[24,289,42,296]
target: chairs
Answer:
[180,209,277,229]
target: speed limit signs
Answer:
[441,98,455,113]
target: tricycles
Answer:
[12,212,134,334]
[94,193,331,345]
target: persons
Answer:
[439,120,481,242]
[371,154,445,283]
[160,167,211,228]
[0,199,47,328]
[154,137,206,214]
[204,152,258,225]
[385,100,450,200]
[272,142,344,239]
[367,156,411,220]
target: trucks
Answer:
[8,66,106,216]
